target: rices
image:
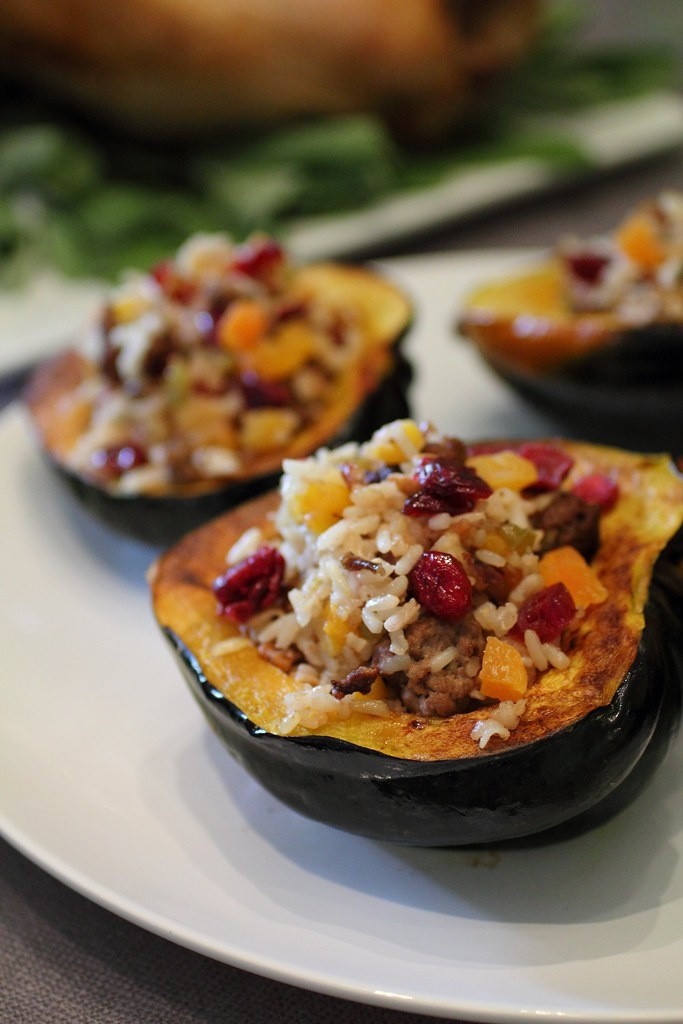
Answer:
[80,224,393,498]
[551,188,682,334]
[210,411,625,747]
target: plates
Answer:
[0,243,681,1019]
[0,3,681,378]
